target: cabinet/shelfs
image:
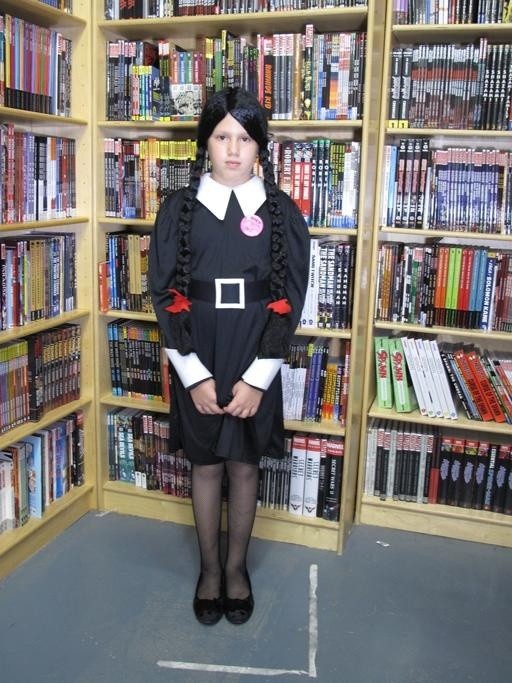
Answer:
[95,0,512,558]
[0,0,96,584]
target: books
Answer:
[0,11,72,117]
[296,236,356,330]
[253,138,361,229]
[215,431,344,522]
[386,1,512,131]
[103,1,368,121]
[1,410,86,531]
[99,231,170,403]
[44,1,73,15]
[1,232,77,331]
[106,407,191,499]
[374,330,512,423]
[1,123,76,224]
[373,139,512,333]
[1,323,81,435]
[365,421,512,517]
[102,136,212,219]
[279,336,350,428]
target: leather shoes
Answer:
[224,564,254,624]
[192,564,225,626]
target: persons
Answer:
[146,87,311,626]
